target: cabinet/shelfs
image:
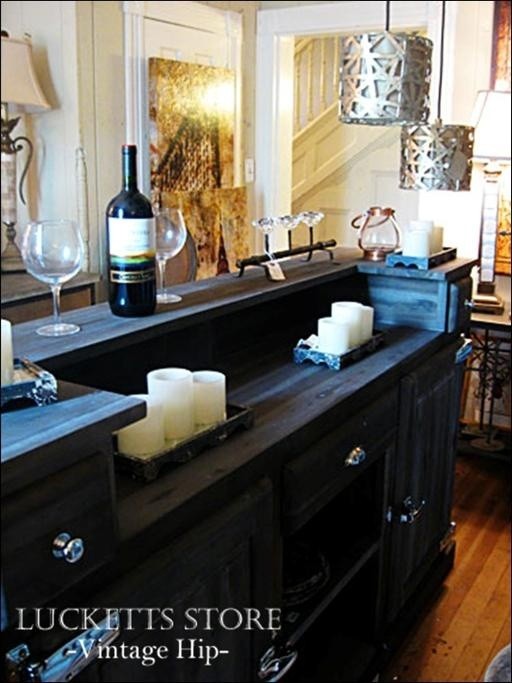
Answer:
[17,480,269,683]
[0,448,116,630]
[273,338,474,681]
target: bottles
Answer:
[104,144,157,318]
[351,206,400,263]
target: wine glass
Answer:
[151,206,188,304]
[21,217,86,337]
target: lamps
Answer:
[336,2,512,329]
[1,28,56,274]
